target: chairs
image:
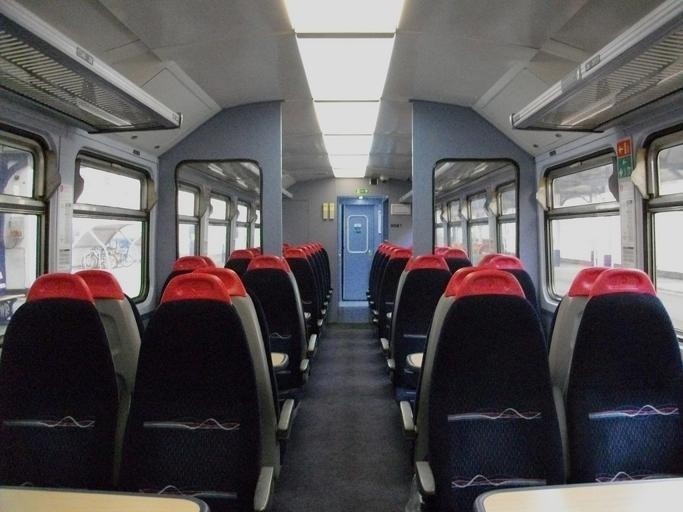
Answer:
[224,240,332,358]
[369,241,542,400]
[75,270,144,493]
[191,268,296,479]
[241,256,308,389]
[0,273,124,494]
[564,269,682,485]
[157,255,208,309]
[417,271,563,512]
[548,267,609,441]
[125,274,280,512]
[413,268,499,461]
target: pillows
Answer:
[0,419,95,487]
[586,407,682,485]
[140,420,242,501]
[446,408,550,489]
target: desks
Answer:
[473,479,683,512]
[271,352,289,371]
[0,486,212,512]
